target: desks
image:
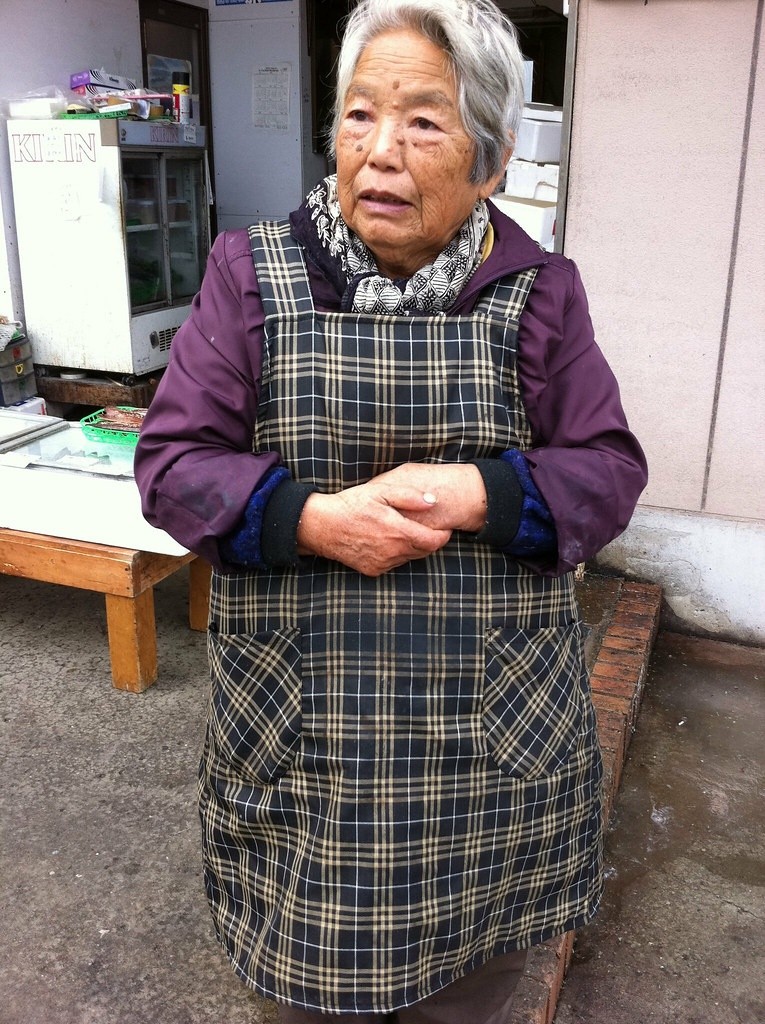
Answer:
[38,375,161,409]
[0,422,211,694]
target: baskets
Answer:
[80,406,140,446]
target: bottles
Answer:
[138,200,154,224]
[172,71,190,125]
[176,200,187,222]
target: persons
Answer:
[134,0,648,1024]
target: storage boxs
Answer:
[512,117,562,163]
[524,61,534,102]
[505,160,559,202]
[0,397,47,415]
[94,88,160,108]
[70,69,137,90]
[489,192,556,245]
[71,85,118,97]
